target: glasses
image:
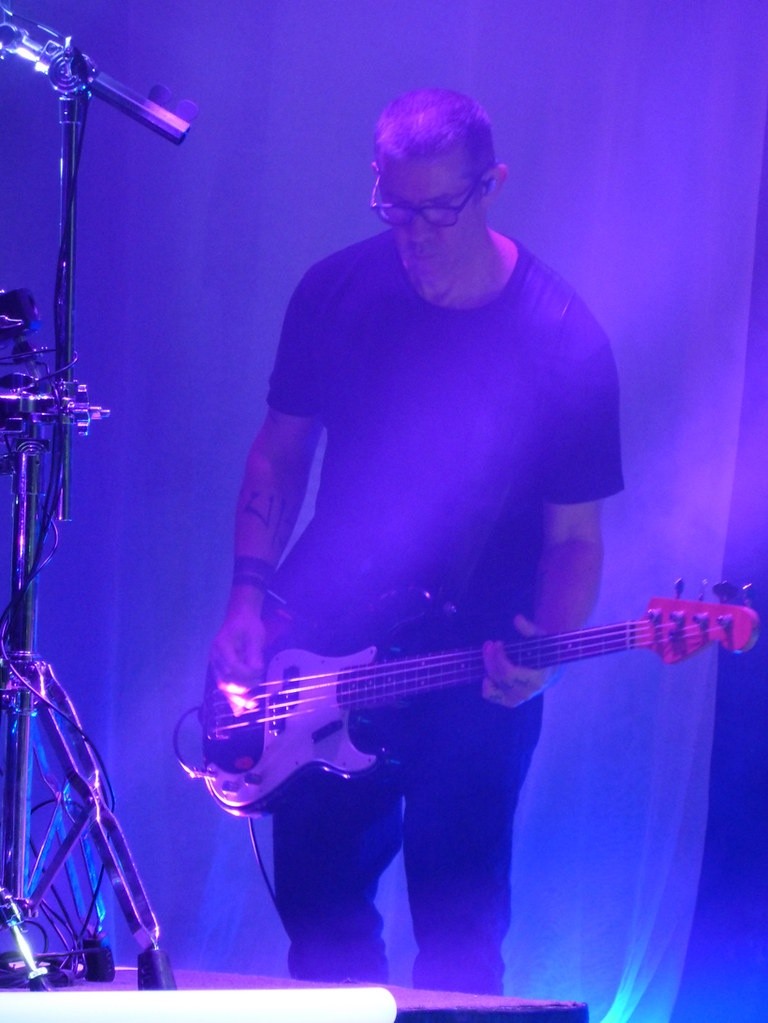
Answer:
[369,168,487,227]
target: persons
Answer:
[209,87,628,998]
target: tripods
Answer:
[1,287,179,991]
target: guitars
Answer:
[203,577,761,818]
